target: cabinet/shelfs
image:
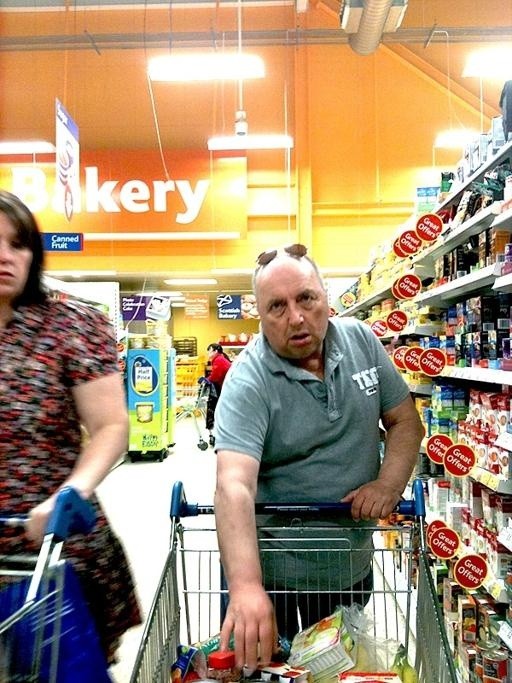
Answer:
[164,350,177,445]
[414,139,512,683]
[127,348,169,452]
[339,230,437,638]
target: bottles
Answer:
[206,651,240,682]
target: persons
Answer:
[210,239,427,674]
[198,342,233,429]
[0,189,144,675]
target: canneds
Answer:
[482,649,508,683]
[474,640,502,679]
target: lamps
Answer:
[420,27,489,151]
[147,0,266,83]
[206,0,297,150]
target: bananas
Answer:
[392,644,417,683]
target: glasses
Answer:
[253,242,310,266]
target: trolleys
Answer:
[129,478,459,681]
[174,377,219,450]
[0,485,95,682]
